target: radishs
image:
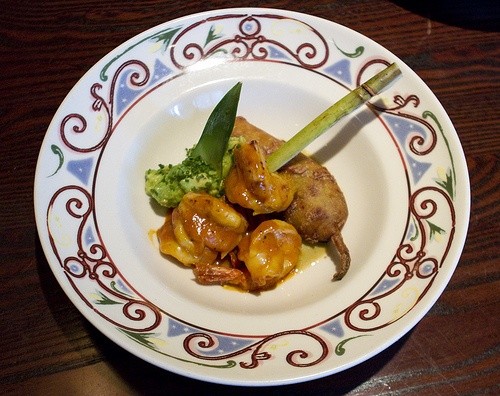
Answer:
[230,115,357,283]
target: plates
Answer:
[34,5,472,389]
